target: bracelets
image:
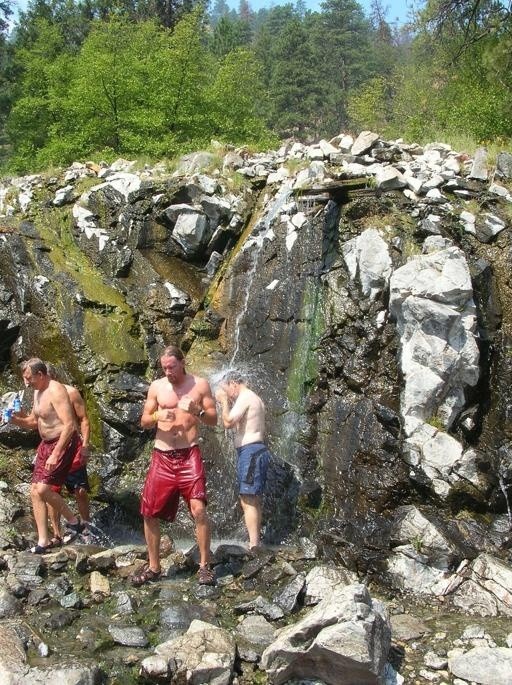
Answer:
[153,413,158,423]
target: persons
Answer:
[132,346,218,586]
[215,372,270,550]
[1,358,87,554]
[44,360,91,548]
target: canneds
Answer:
[4,408,12,423]
[14,399,22,412]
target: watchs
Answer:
[197,408,205,419]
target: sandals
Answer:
[130,566,162,586]
[63,519,86,545]
[26,545,52,555]
[198,568,215,585]
[48,536,63,548]
